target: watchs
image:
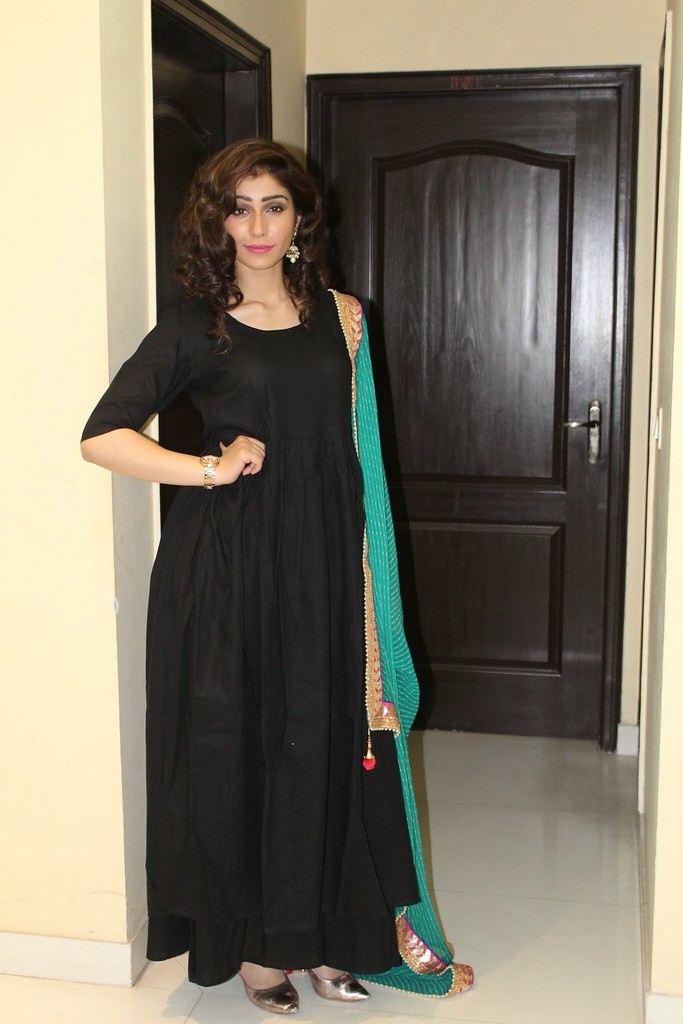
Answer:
[200,455,219,489]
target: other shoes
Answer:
[299,968,372,1001]
[238,968,300,1014]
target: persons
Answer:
[81,138,474,1015]
